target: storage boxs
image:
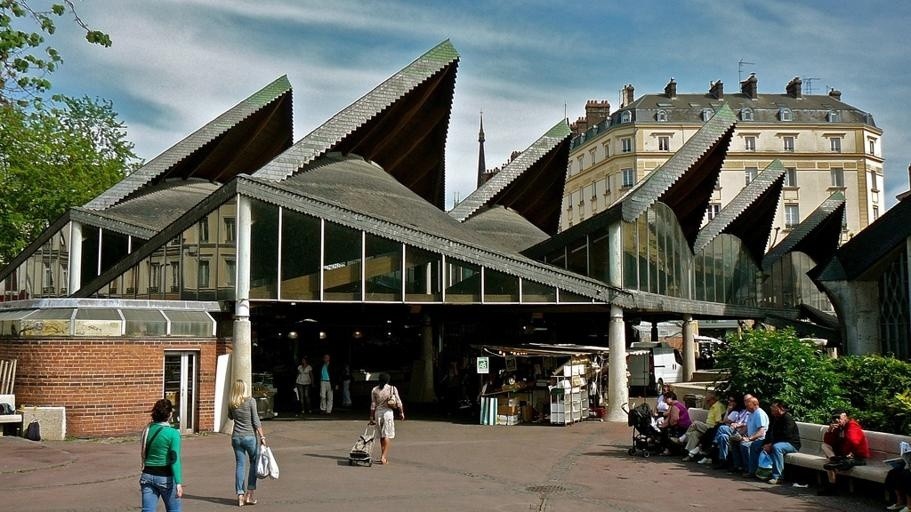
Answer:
[497,397,532,426]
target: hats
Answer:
[830,409,844,414]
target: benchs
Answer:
[687,407,911,502]
[0,394,22,436]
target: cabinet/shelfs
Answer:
[508,388,549,404]
[252,371,278,420]
[549,353,610,426]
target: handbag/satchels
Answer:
[26,422,41,440]
[257,444,269,480]
[388,395,397,409]
[266,447,279,480]
[0,403,15,415]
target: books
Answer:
[882,442,911,467]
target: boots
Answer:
[824,457,841,470]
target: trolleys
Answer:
[348,423,378,466]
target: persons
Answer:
[654,385,801,484]
[821,409,871,472]
[295,355,315,415]
[317,353,340,415]
[138,398,184,512]
[887,469,911,512]
[369,373,404,465]
[341,363,352,407]
[227,378,265,507]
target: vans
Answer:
[626,342,685,395]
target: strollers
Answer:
[621,402,666,457]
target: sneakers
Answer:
[660,437,784,484]
[887,504,905,509]
[899,506,911,512]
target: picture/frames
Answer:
[504,356,517,372]
[477,356,490,374]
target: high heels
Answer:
[239,495,258,507]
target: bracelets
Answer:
[259,435,266,440]
